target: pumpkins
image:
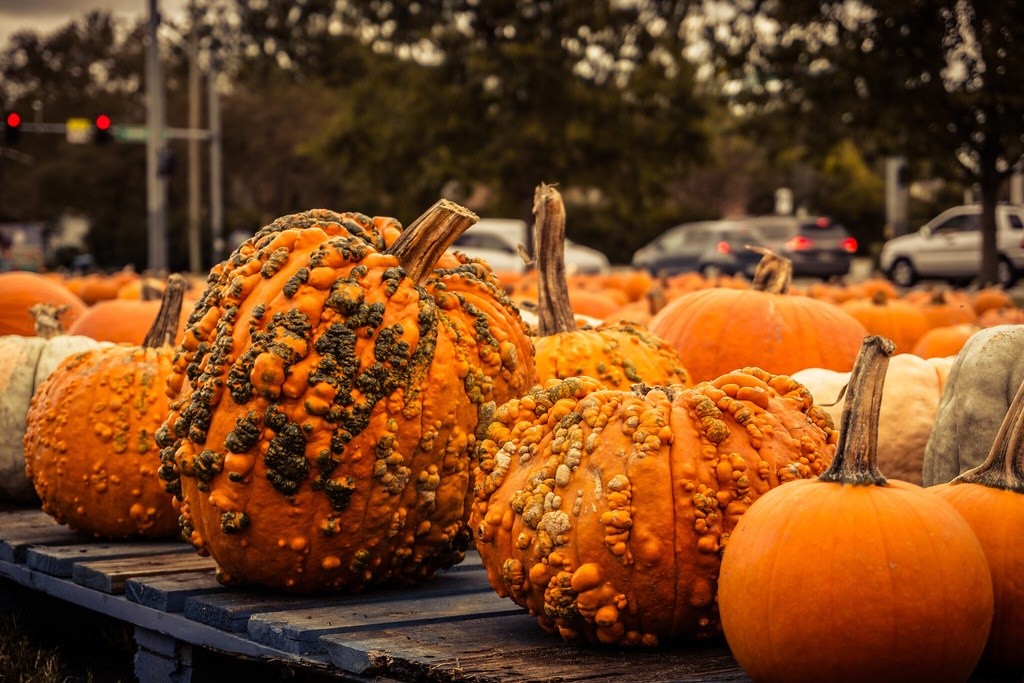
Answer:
[0,180,1024,683]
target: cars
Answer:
[751,216,860,283]
[444,217,611,283]
[631,213,772,281]
[881,199,1024,290]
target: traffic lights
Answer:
[5,113,21,140]
[96,114,112,146]
